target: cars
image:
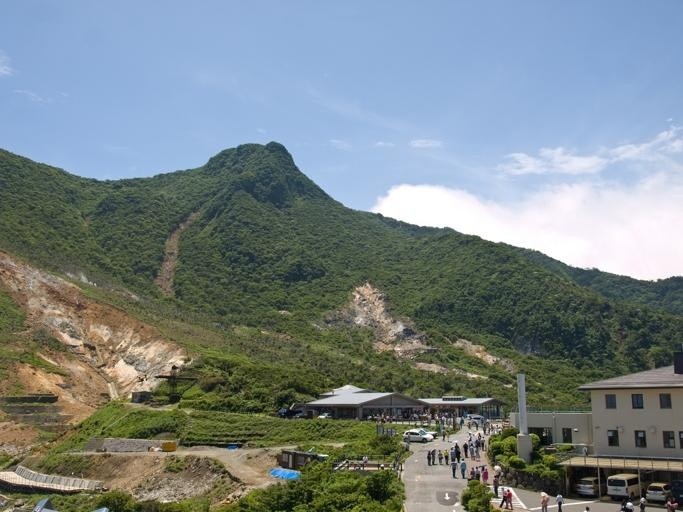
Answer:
[470,416,490,425]
[317,413,331,420]
[402,429,438,442]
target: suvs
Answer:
[646,482,673,503]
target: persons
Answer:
[368,411,514,512]
[556,492,563,512]
[540,491,550,512]
[625,497,634,512]
[639,494,647,512]
[584,507,590,512]
[667,496,678,512]
[671,497,676,512]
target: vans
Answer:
[607,473,645,501]
[576,477,606,497]
